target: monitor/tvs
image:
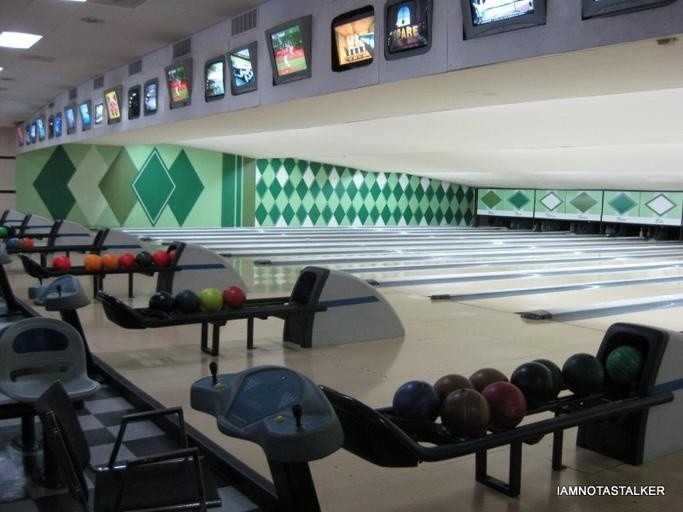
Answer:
[16,0,683,147]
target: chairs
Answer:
[0,263,101,453]
